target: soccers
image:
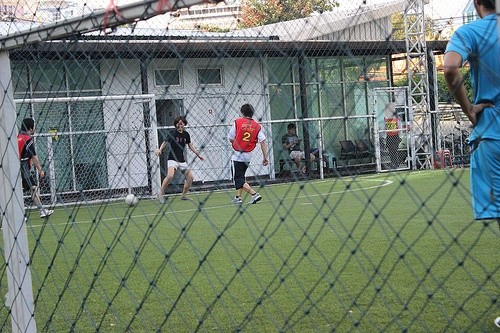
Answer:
[125,193,138,206]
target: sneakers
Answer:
[232,196,242,204]
[248,193,262,204]
[40,209,54,218]
[25,217,28,222]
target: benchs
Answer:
[279,156,330,177]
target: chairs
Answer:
[338,139,382,169]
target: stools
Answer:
[435,150,453,169]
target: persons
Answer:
[443,0,500,329]
[381,103,403,168]
[281,123,315,179]
[17,118,55,222]
[228,104,269,204]
[153,116,205,204]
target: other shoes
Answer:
[494,316,500,327]
[181,196,192,200]
[159,195,164,204]
